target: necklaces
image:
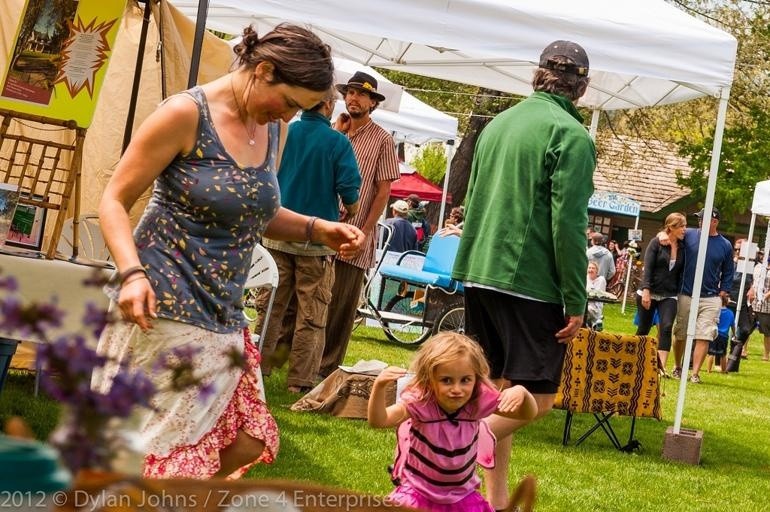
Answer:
[229,76,258,146]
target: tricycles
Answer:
[346,224,479,350]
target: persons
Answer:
[378,193,766,383]
[89,20,366,480]
[451,40,599,512]
[366,331,540,510]
[257,78,363,395]
[273,72,400,376]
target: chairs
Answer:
[553,330,661,453]
[242,241,278,353]
[34,213,114,397]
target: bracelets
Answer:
[118,264,150,282]
[303,212,321,249]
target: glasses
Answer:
[734,248,740,251]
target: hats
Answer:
[449,207,464,219]
[403,194,420,203]
[693,207,720,221]
[390,200,409,213]
[539,40,589,76]
[335,71,385,102]
[757,248,770,258]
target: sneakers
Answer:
[660,368,669,377]
[689,373,700,383]
[671,366,682,378]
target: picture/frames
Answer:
[4,191,49,251]
